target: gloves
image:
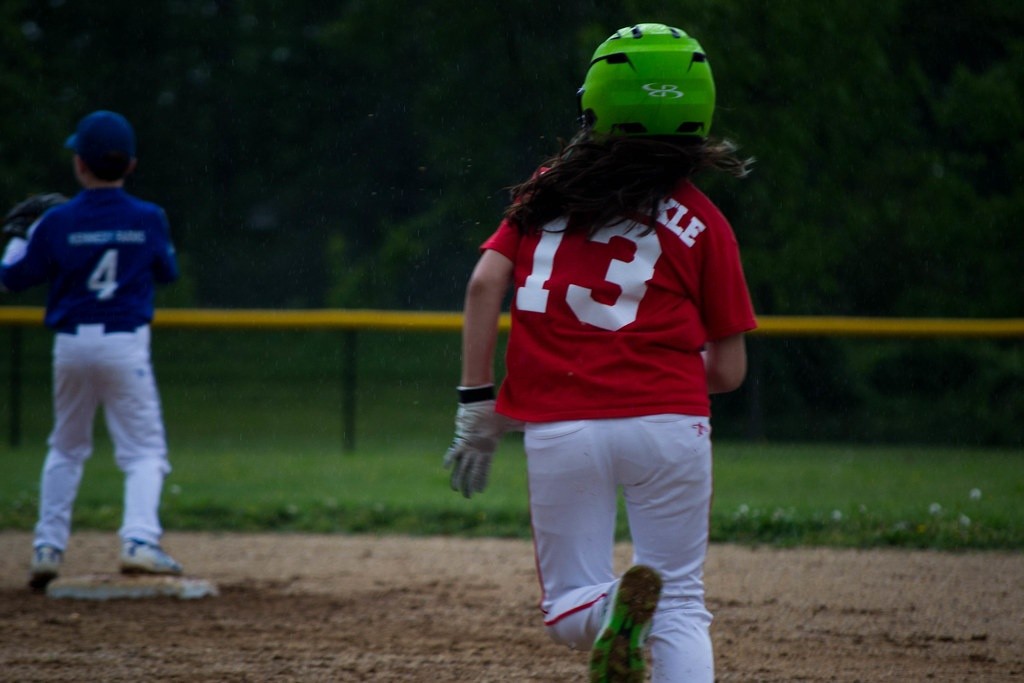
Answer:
[442,384,502,498]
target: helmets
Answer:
[577,24,716,138]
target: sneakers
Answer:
[24,543,61,593]
[118,541,184,575]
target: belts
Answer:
[56,323,138,335]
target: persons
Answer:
[0,112,184,592]
[442,23,757,683]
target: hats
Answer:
[65,111,136,166]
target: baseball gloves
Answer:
[0,192,69,234]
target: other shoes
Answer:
[588,565,663,683]
